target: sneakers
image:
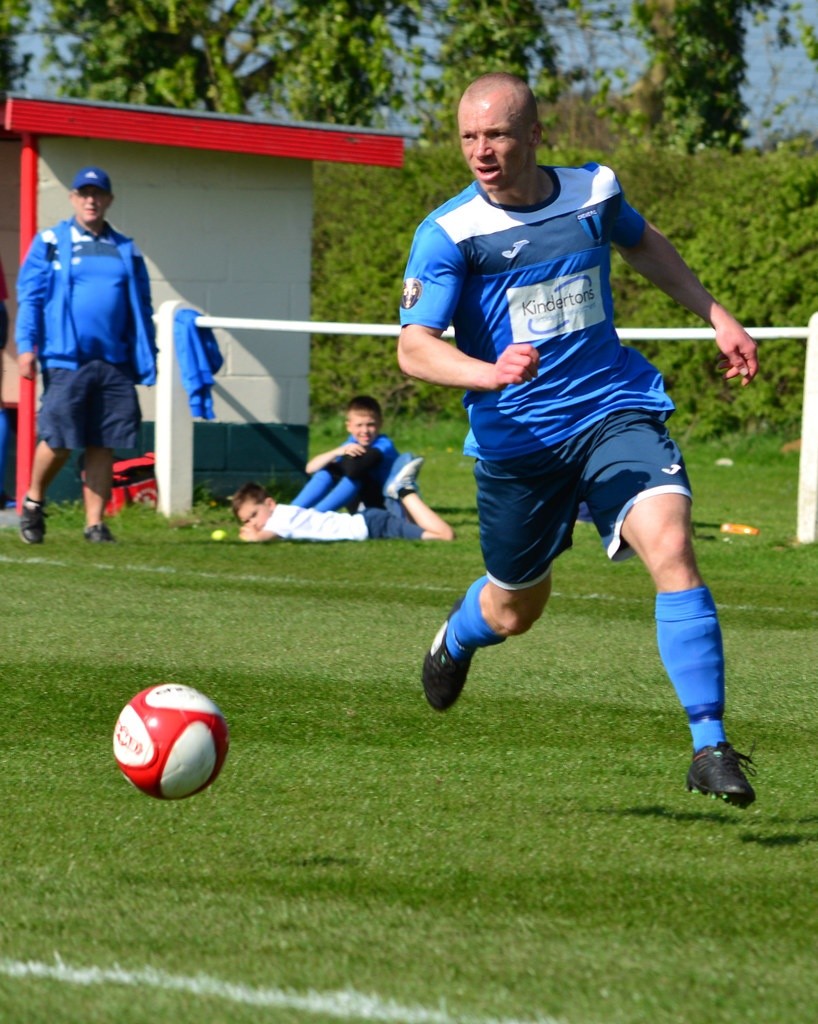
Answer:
[18,494,50,545]
[386,456,424,500]
[83,523,114,544]
[687,741,757,808]
[421,599,478,712]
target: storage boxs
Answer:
[79,453,160,518]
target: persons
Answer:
[230,455,456,540]
[292,394,403,514]
[13,162,160,543]
[395,72,761,804]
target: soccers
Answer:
[112,683,229,801]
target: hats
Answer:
[71,165,112,194]
